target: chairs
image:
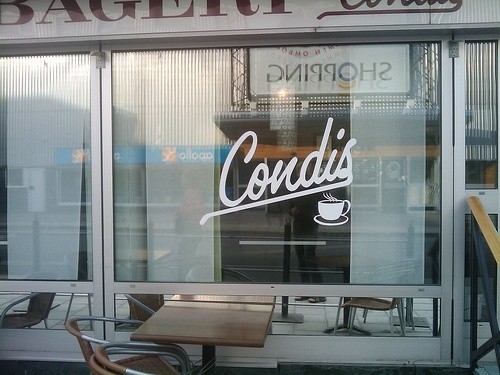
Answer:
[339,296,406,337]
[1,292,54,329]
[89,347,195,375]
[465,195,500,371]
[65,315,144,371]
[94,342,194,375]
[122,293,164,330]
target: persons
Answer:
[286,160,329,304]
[170,163,206,291]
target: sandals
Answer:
[295,296,310,301]
[309,296,327,303]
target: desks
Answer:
[130,301,275,375]
[113,246,174,281]
